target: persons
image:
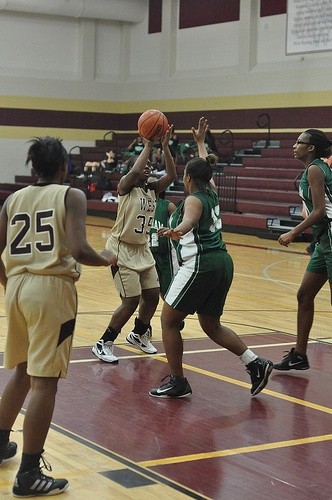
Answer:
[0,136,118,497]
[77,116,332,398]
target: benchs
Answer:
[0,131,332,243]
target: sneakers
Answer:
[148,375,193,399]
[244,357,273,396]
[305,243,315,255]
[125,327,158,354]
[12,469,69,497]
[273,348,310,371]
[91,339,119,363]
[0,441,18,460]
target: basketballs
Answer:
[137,109,170,141]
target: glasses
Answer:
[295,139,316,146]
